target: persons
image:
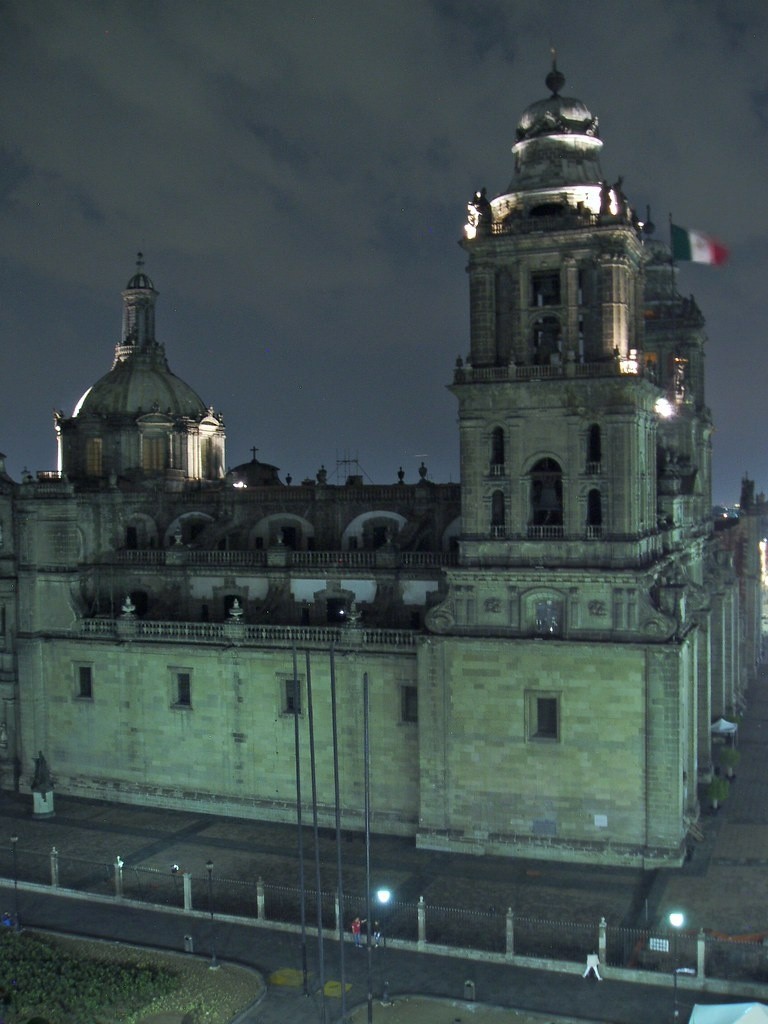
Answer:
[351,916,368,948]
[582,949,604,982]
[373,921,382,948]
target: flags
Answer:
[670,223,729,265]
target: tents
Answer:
[710,718,739,752]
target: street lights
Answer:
[376,887,391,1008]
[668,912,683,1024]
[205,860,221,968]
[10,832,19,921]
